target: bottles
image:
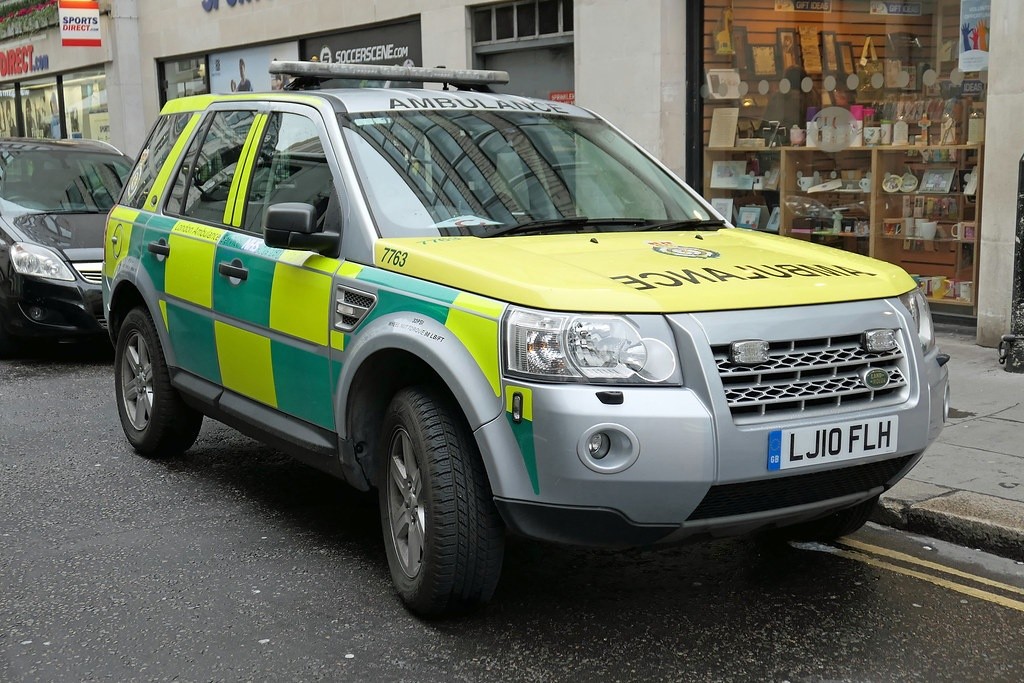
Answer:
[806,107,819,147]
[880,119,892,145]
[848,105,876,147]
[968,107,984,144]
[894,115,908,145]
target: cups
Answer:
[901,217,914,236]
[909,274,973,301]
[836,125,846,144]
[790,124,807,146]
[821,125,835,144]
[863,126,886,146]
[882,217,904,236]
[951,222,975,240]
[854,219,868,236]
[796,176,822,191]
[858,178,870,192]
[915,218,937,240]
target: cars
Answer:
[0,134,141,358]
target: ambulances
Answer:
[100,60,952,617]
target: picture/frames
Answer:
[730,27,857,77]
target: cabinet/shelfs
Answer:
[702,144,983,307]
